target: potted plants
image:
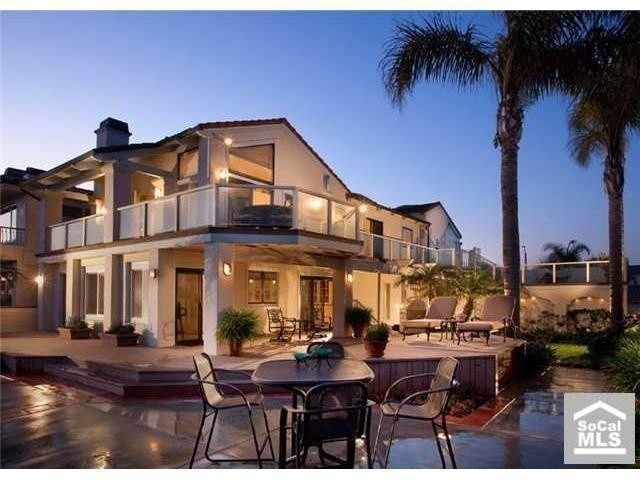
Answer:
[215,306,263,357]
[55,315,92,340]
[96,324,139,347]
[361,321,391,357]
[345,303,374,341]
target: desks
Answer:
[251,358,375,469]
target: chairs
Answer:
[400,296,456,344]
[266,307,332,346]
[292,342,353,454]
[457,295,517,346]
[276,383,375,468]
[189,352,275,469]
[372,356,462,470]
